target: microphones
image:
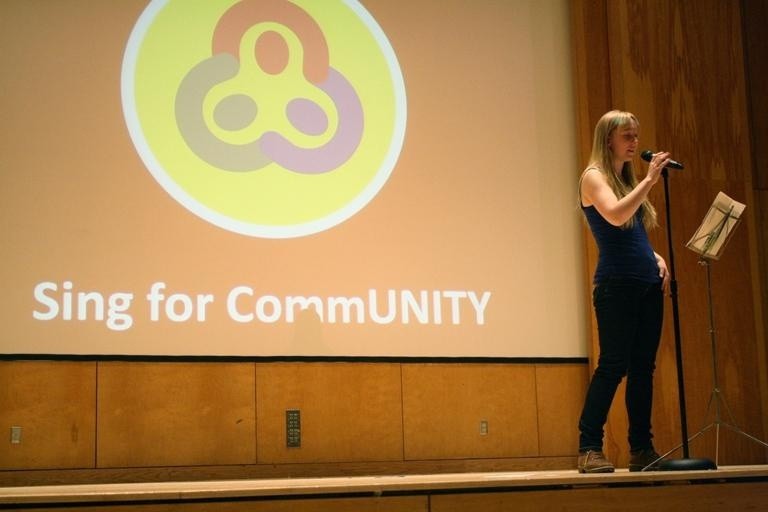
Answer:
[641,150,684,170]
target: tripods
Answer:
[641,259,768,473]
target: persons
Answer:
[576,108,673,478]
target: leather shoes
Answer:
[629,450,668,471]
[577,450,614,473]
